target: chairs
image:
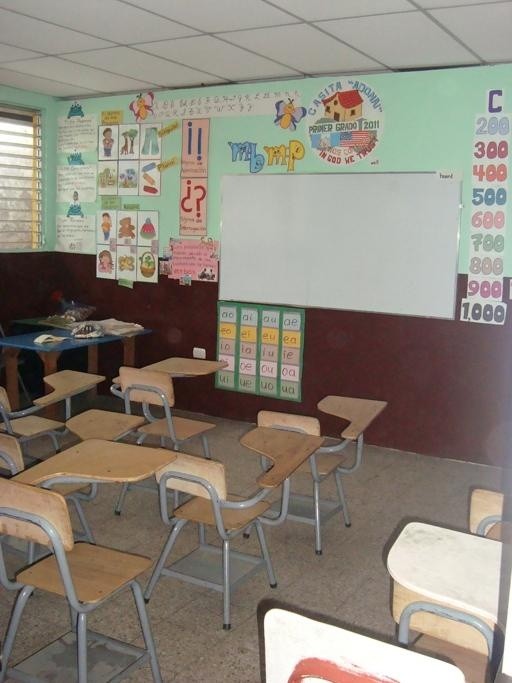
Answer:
[0,474,161,682]
[256,409,354,557]
[0,382,69,450]
[264,608,468,683]
[0,428,95,566]
[467,486,511,539]
[116,364,214,459]
[143,444,278,631]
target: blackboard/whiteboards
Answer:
[217,170,462,321]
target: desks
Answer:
[1,318,151,413]
